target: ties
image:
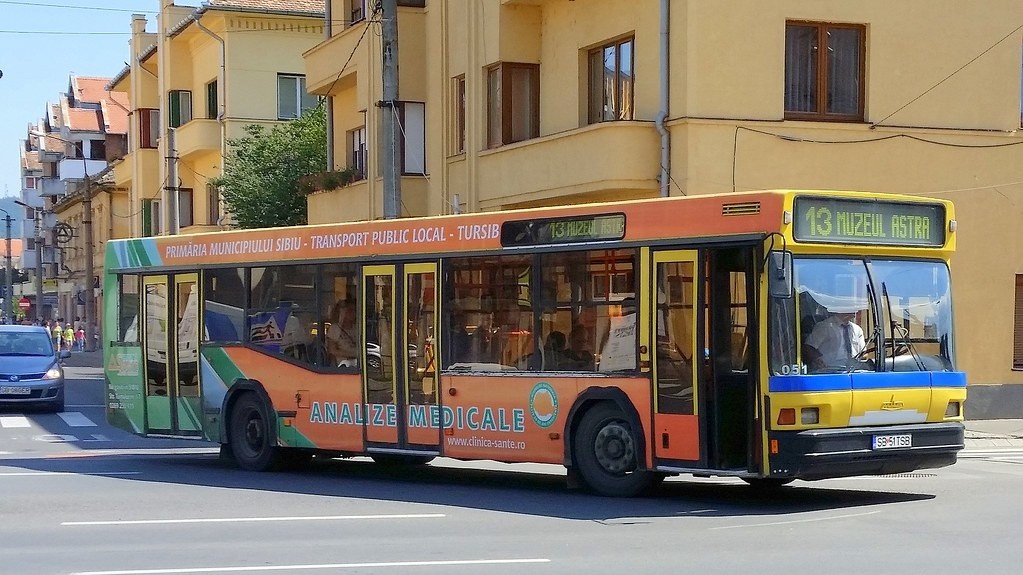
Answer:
[842,325,852,358]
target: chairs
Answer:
[294,322,332,364]
[802,313,832,337]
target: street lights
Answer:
[14,201,44,324]
[30,130,96,351]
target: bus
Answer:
[102,190,967,498]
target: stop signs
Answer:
[18,298,31,311]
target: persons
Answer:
[51,321,64,352]
[34,318,57,351]
[322,299,358,368]
[621,297,637,316]
[280,304,316,363]
[802,302,868,371]
[527,331,590,371]
[73,317,81,339]
[56,317,67,350]
[63,323,76,351]
[469,312,502,365]
[74,326,87,352]
[451,312,472,363]
[80,316,87,336]
[563,323,595,371]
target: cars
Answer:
[0,324,71,412]
